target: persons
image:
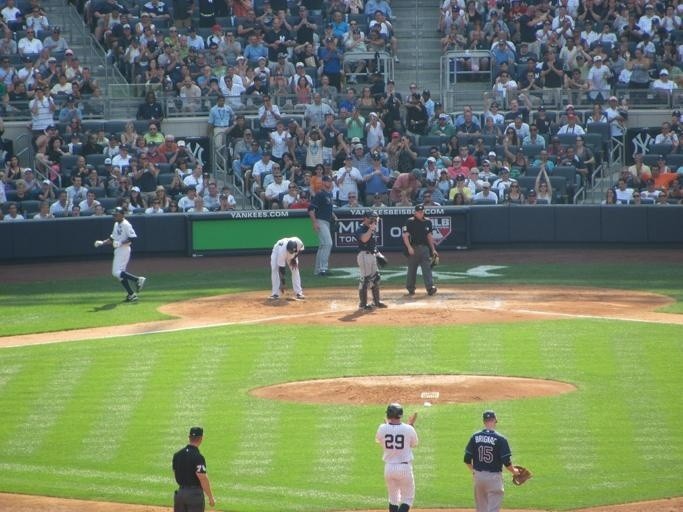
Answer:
[92,205,147,302]
[373,401,419,511]
[462,409,520,511]
[401,204,440,297]
[169,425,216,511]
[265,236,307,303]
[352,210,389,312]
[306,175,340,277]
[1,0,683,224]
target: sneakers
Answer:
[123,292,136,304]
[137,276,146,291]
[297,294,305,299]
[358,304,373,310]
[267,294,279,300]
[427,287,436,295]
[376,302,388,307]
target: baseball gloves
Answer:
[376,252,389,268]
[512,465,532,486]
[431,254,440,267]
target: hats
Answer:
[190,428,203,436]
[322,175,335,181]
[415,204,423,210]
[483,410,497,424]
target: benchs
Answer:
[0,0,683,218]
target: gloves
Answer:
[95,240,103,248]
[113,242,119,249]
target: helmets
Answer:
[384,404,402,419]
[111,206,122,216]
[366,211,379,225]
[287,241,297,253]
